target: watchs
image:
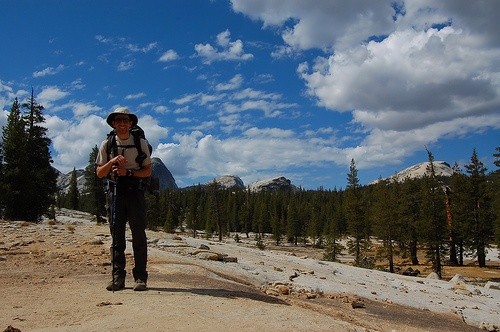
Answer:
[130,170,136,176]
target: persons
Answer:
[95,108,152,291]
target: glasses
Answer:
[114,118,131,123]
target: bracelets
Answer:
[125,169,130,176]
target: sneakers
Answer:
[133,280,146,290]
[106,278,125,290]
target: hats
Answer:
[107,107,139,128]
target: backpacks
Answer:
[105,124,152,169]
